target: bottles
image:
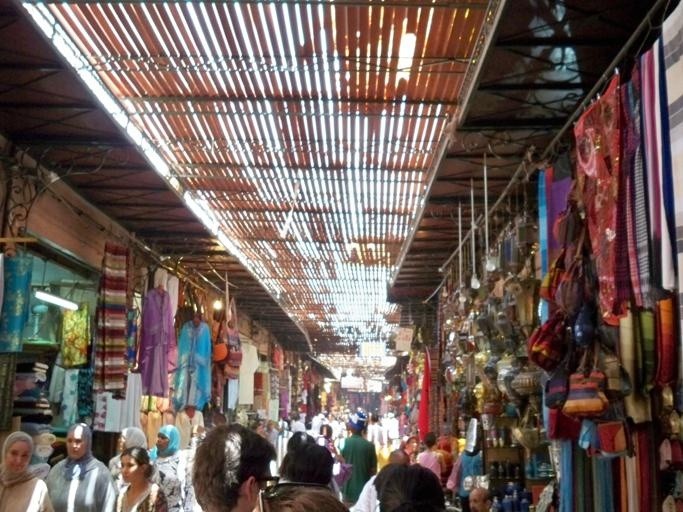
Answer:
[485,425,533,512]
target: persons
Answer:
[469,486,492,511]
[460,442,481,512]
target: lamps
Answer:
[456,150,497,304]
[32,255,80,311]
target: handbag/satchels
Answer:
[528,179,636,428]
